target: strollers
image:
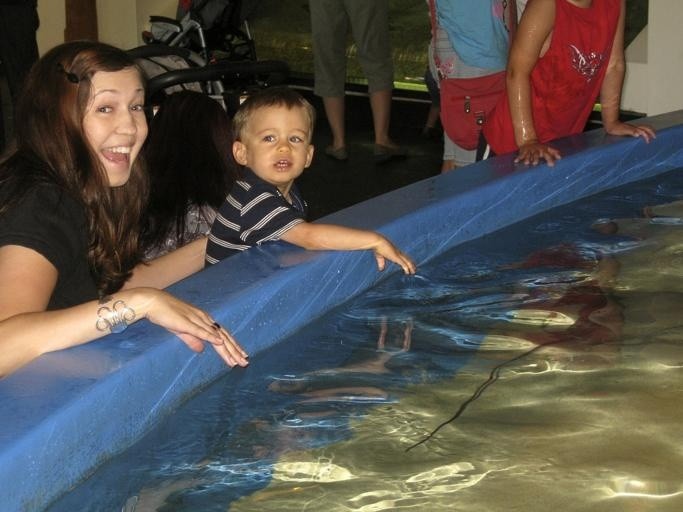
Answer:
[124,2,287,116]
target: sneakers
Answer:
[373,142,417,161]
[324,144,349,162]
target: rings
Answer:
[210,320,222,331]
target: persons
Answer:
[0,39,250,380]
[426,1,518,173]
[307,0,409,163]
[420,42,440,139]
[203,83,417,277]
[142,89,242,261]
[506,0,657,167]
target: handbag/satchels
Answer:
[441,70,506,151]
